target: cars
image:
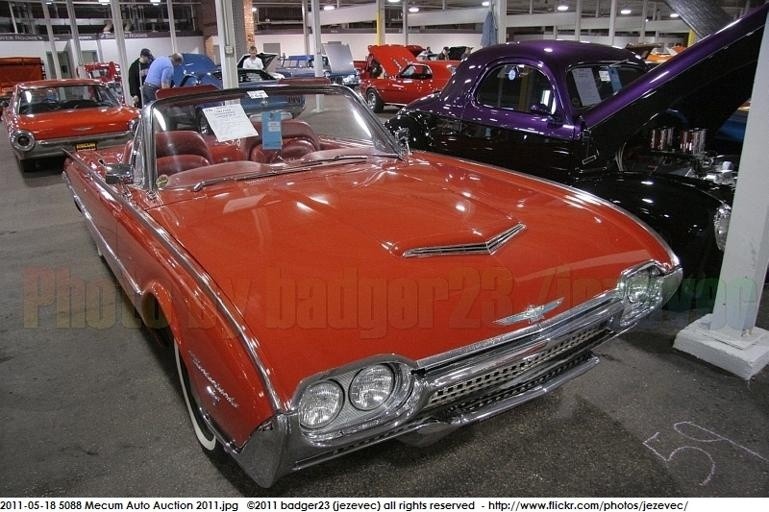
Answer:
[61,78,684,489]
[385,1,769,267]
[0,77,141,174]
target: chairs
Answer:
[124,130,212,183]
[239,121,326,162]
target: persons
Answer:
[128,48,153,108]
[463,46,472,59]
[242,46,264,82]
[436,47,450,61]
[141,52,184,104]
[419,46,433,61]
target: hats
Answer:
[141,49,153,59]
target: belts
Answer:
[143,81,159,90]
